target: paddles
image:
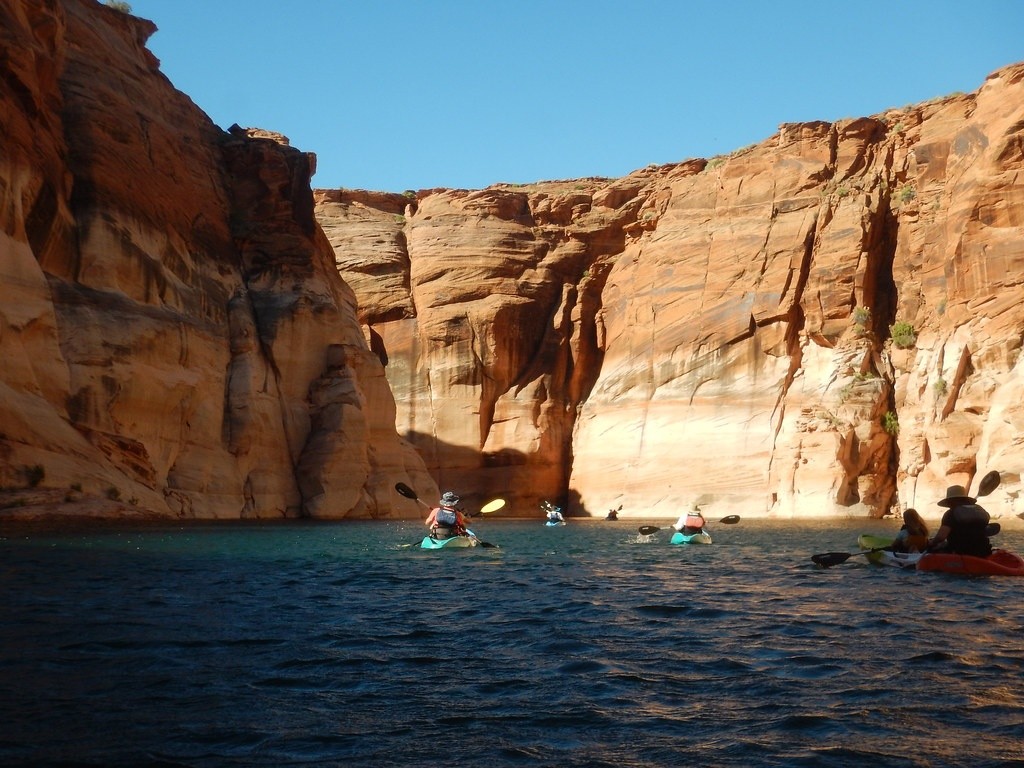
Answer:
[617,505,624,511]
[638,515,741,536]
[539,500,552,513]
[401,498,506,548]
[395,482,498,548]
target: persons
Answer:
[929,485,993,557]
[547,505,565,522]
[426,491,472,541]
[892,508,930,553]
[672,505,705,536]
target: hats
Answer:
[548,506,561,511]
[937,485,977,508]
[439,492,459,506]
[685,502,701,513]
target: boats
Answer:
[606,516,618,521]
[670,529,712,545]
[546,520,566,528]
[857,533,1024,577]
[421,528,477,549]
